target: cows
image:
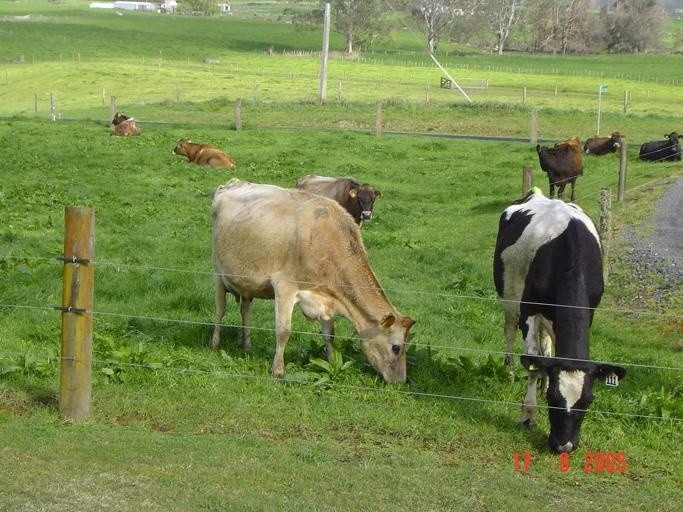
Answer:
[112,112,140,136]
[492,186,627,454]
[171,139,237,169]
[584,132,624,156]
[296,174,382,229]
[639,132,683,163]
[209,177,417,387]
[536,136,585,208]
